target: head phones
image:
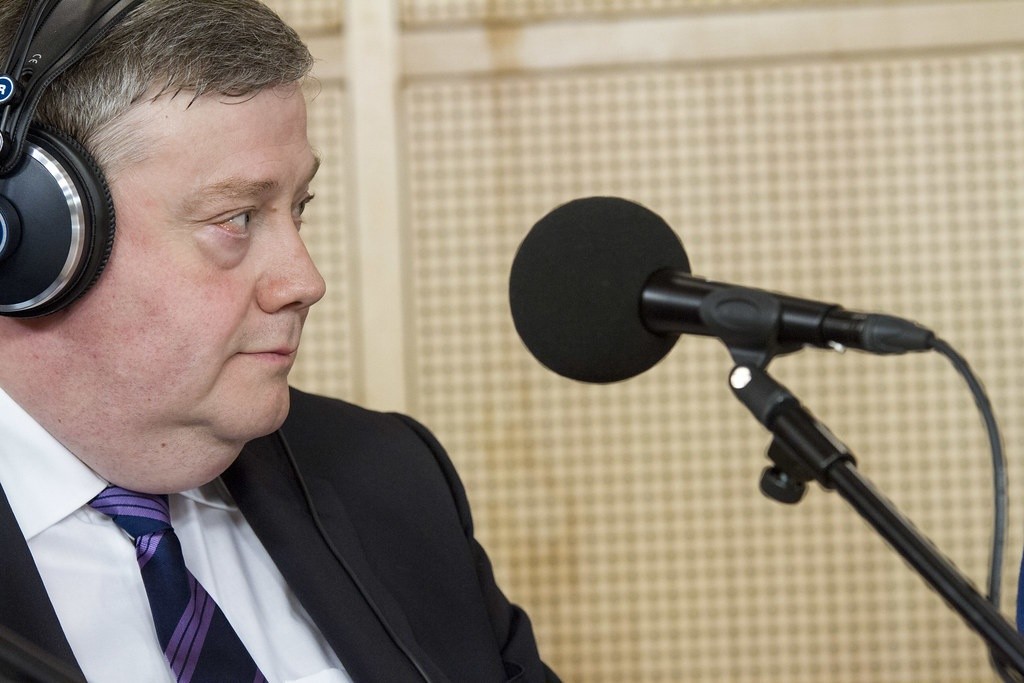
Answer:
[0,0,143,319]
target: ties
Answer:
[87,487,269,683]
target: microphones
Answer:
[506,196,934,386]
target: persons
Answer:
[0,0,560,683]
